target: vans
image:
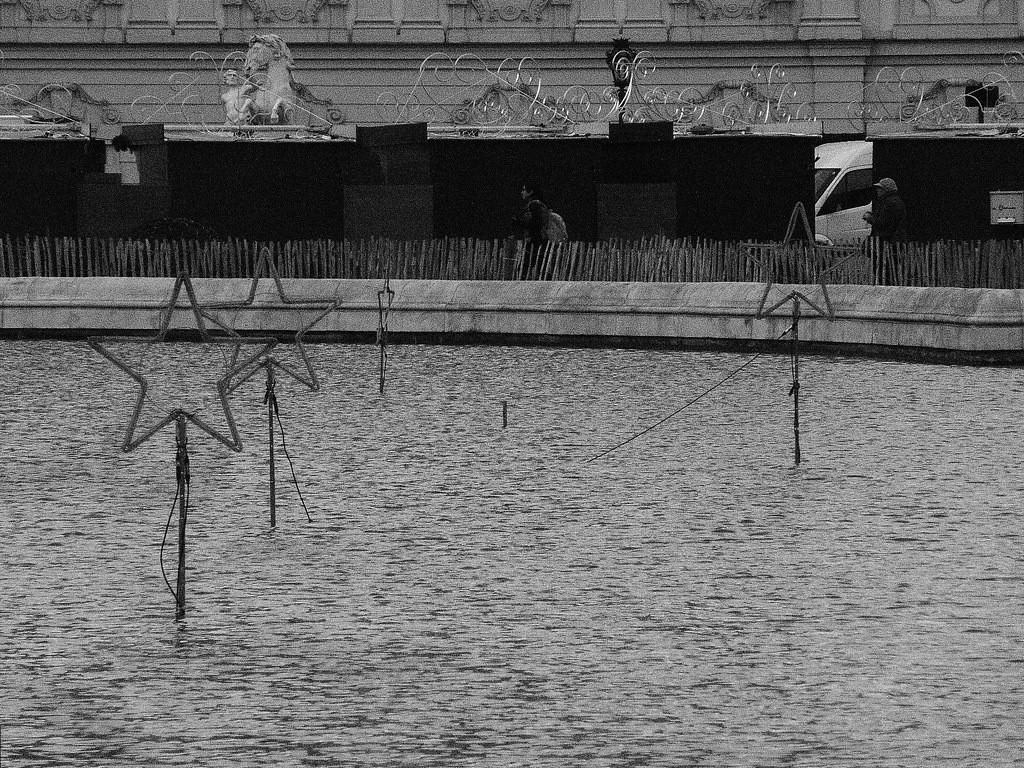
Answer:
[812,140,873,273]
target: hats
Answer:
[873,178,898,194]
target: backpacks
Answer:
[536,200,569,246]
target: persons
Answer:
[517,183,548,281]
[864,178,907,287]
[221,70,255,125]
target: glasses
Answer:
[521,188,526,192]
